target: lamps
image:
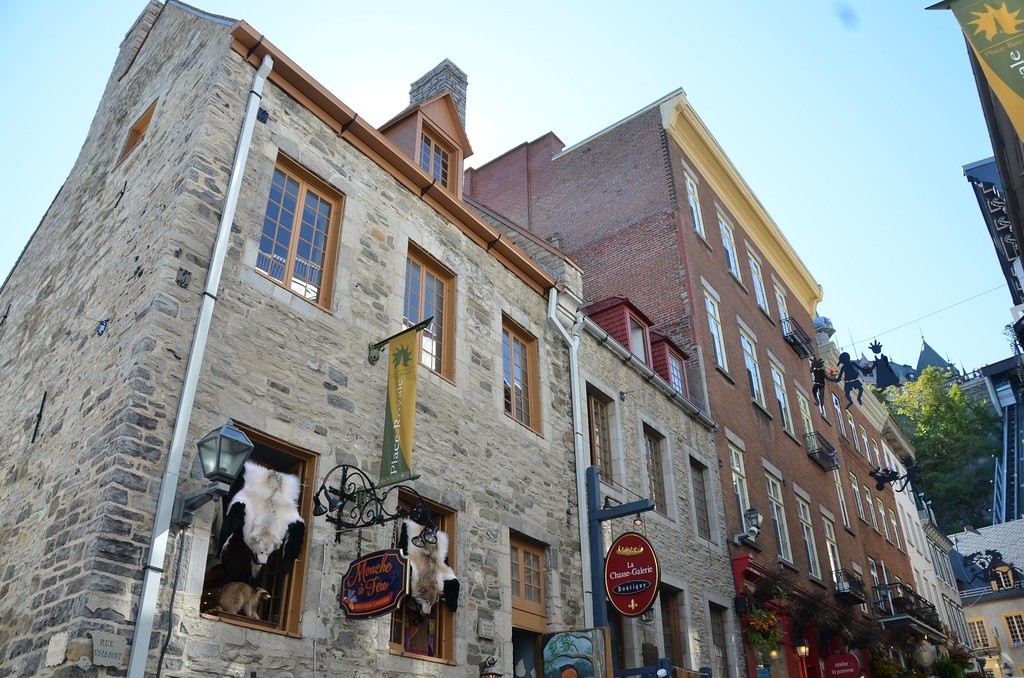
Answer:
[767,639,785,663]
[313,463,440,548]
[170,418,256,530]
[478,654,505,678]
[792,636,810,658]
[737,503,764,544]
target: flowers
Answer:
[743,603,785,652]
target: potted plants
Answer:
[892,596,943,634]
[838,578,865,602]
